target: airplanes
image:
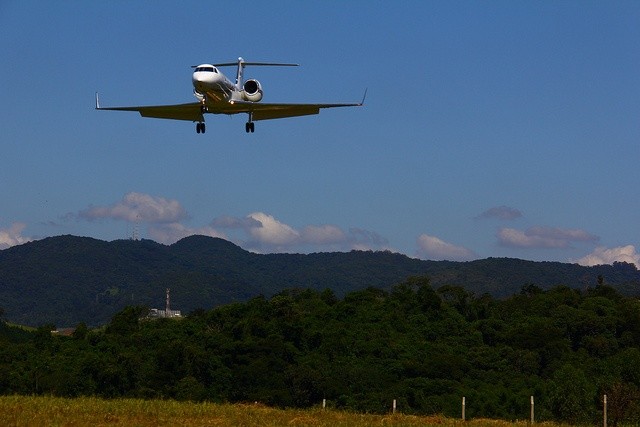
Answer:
[94,55,369,135]
